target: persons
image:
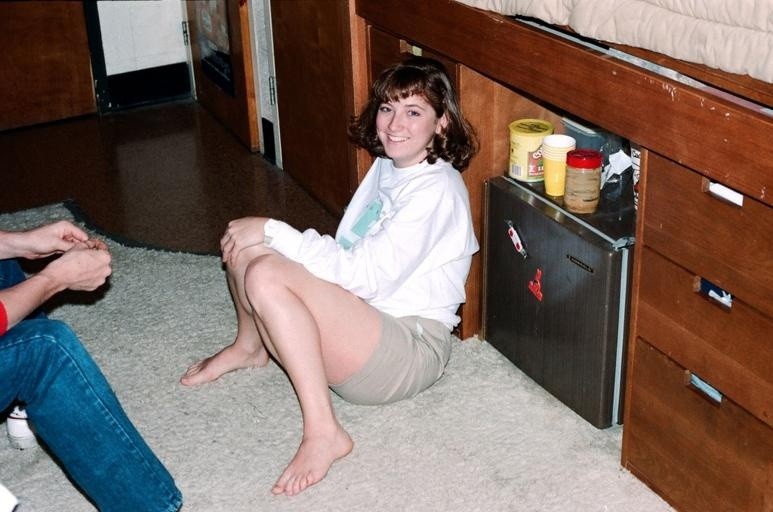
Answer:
[180,49,489,498]
[0,219,185,512]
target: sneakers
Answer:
[6,405,44,451]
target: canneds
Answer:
[510,119,554,182]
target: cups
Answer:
[539,135,577,198]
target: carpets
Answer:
[0,199,675,512]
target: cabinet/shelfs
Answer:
[270,0,773,512]
